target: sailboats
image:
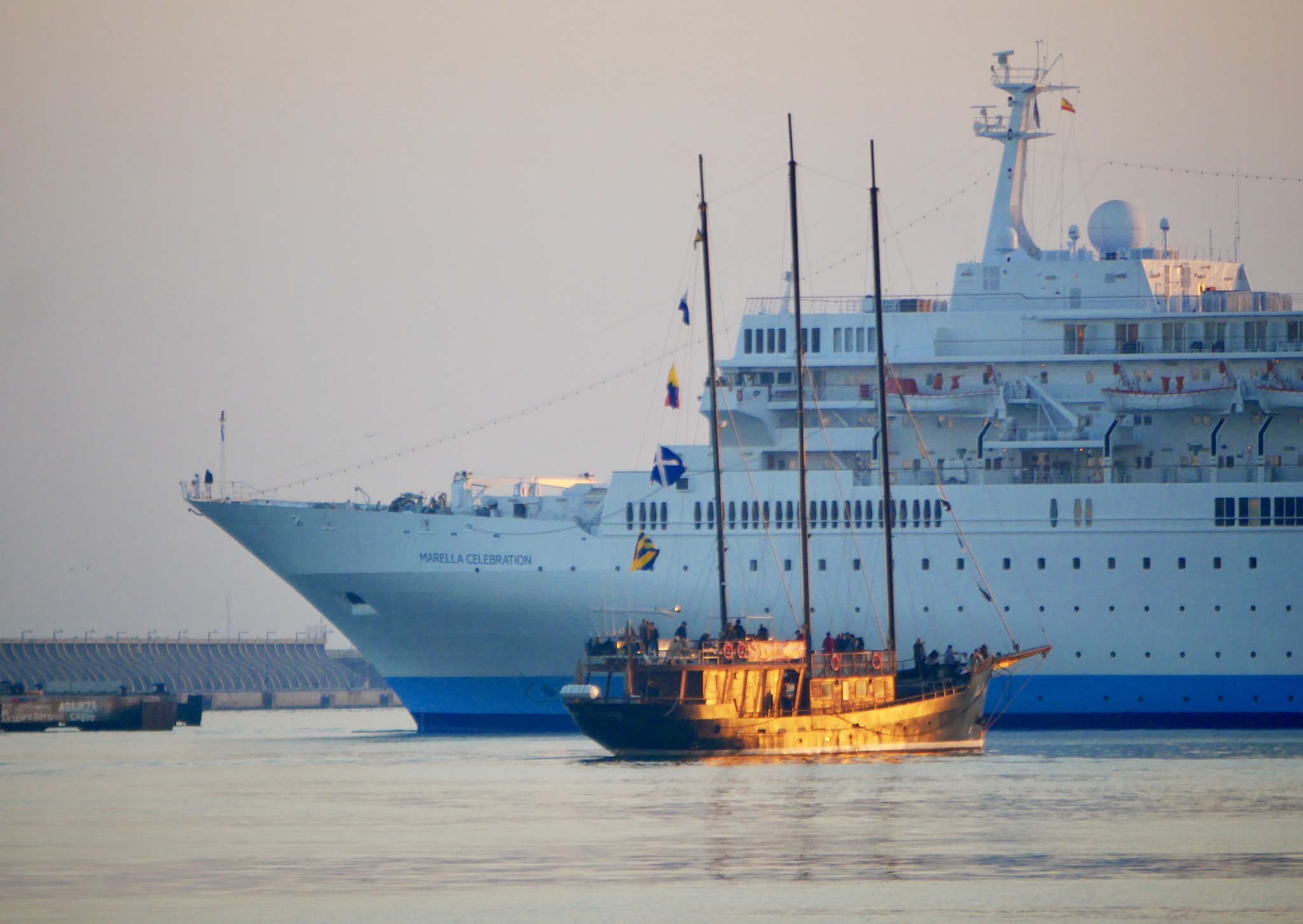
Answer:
[559,113,1053,757]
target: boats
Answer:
[180,39,1303,735]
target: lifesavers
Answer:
[722,643,733,660]
[736,643,747,660]
[888,472,898,485]
[871,653,881,670]
[830,654,839,671]
[1092,470,1103,484]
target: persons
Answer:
[675,621,694,664]
[944,645,956,676]
[791,630,806,663]
[698,631,715,649]
[762,689,773,716]
[755,624,769,641]
[914,638,926,679]
[205,469,213,499]
[779,686,793,714]
[720,619,746,663]
[969,644,988,676]
[625,619,660,662]
[822,632,864,675]
[192,474,200,498]
[586,636,617,656]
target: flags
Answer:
[629,528,660,572]
[693,228,702,250]
[1033,95,1040,129]
[650,444,688,487]
[664,363,680,410]
[1061,96,1076,113]
[678,291,690,326]
